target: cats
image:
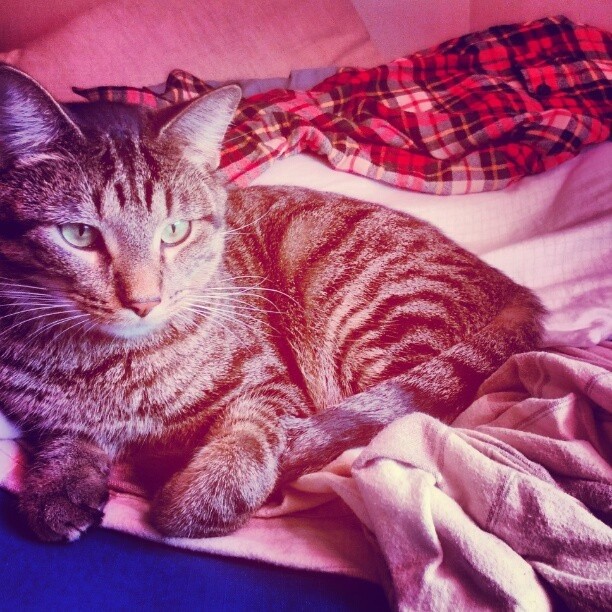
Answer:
[0,61,547,544]
